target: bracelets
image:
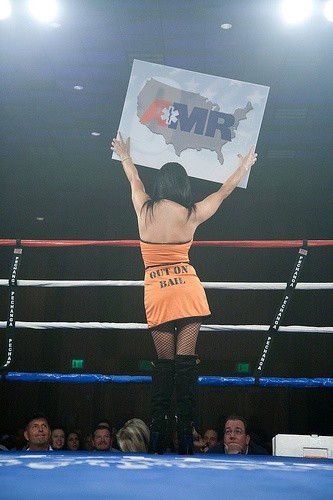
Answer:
[121,157,132,162]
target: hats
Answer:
[22,412,49,430]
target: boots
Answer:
[175,355,197,455]
[149,358,176,454]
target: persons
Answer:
[24,417,53,451]
[111,131,258,455]
[205,415,268,454]
[0,421,112,451]
[116,418,150,452]
[90,426,120,451]
[148,416,210,454]
[203,430,221,449]
